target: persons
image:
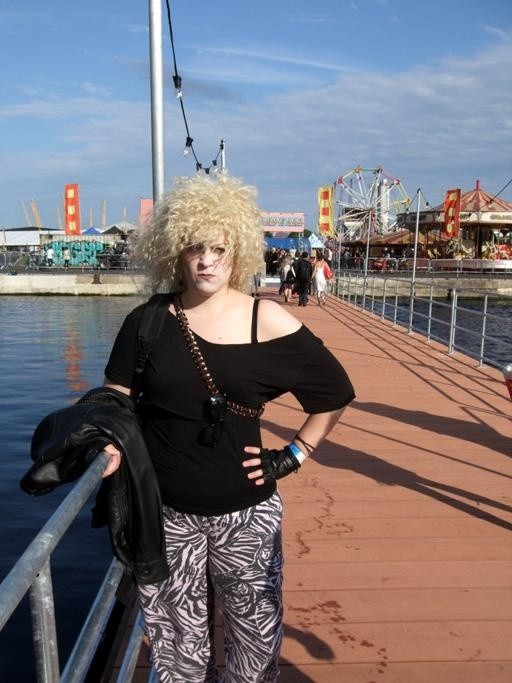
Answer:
[261,243,334,308]
[96,162,357,682]
[334,228,512,276]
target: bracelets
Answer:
[288,441,305,464]
[294,433,316,456]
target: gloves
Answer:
[258,445,301,484]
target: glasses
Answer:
[197,390,226,450]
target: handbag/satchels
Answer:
[323,260,332,279]
[286,265,296,284]
